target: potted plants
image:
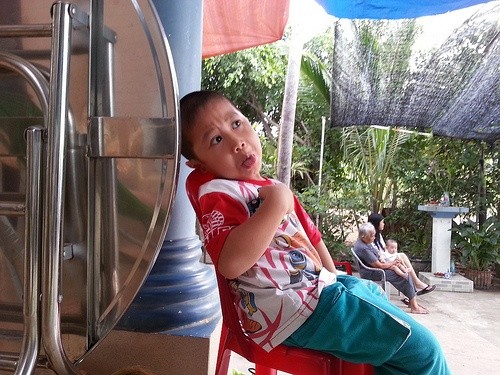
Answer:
[448,215,500,288]
[401,220,431,272]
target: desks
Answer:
[0,0,182,374]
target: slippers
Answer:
[404,298,409,305]
[411,307,427,314]
[417,285,435,295]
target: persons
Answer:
[368,213,435,305]
[379,239,413,278]
[178,91,449,375]
[353,222,431,314]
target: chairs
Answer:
[351,248,386,292]
[185,169,375,375]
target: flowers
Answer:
[427,160,452,193]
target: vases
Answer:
[439,192,450,207]
